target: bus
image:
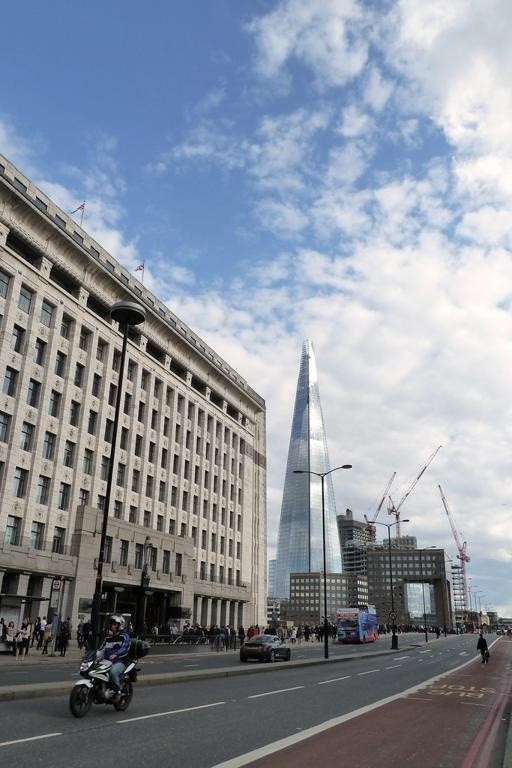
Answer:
[336,605,379,644]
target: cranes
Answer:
[364,445,442,546]
[439,483,475,634]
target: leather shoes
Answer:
[21,657,24,661]
[15,657,18,661]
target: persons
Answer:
[140,621,338,650]
[376,622,457,634]
[96,613,133,705]
[443,624,448,637]
[0,615,94,662]
[435,624,441,638]
[476,633,487,665]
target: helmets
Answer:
[108,614,125,635]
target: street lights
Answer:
[292,465,352,658]
[404,546,437,643]
[432,560,493,639]
[368,520,410,650]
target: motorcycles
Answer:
[69,640,151,718]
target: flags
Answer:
[71,202,85,216]
[134,264,144,273]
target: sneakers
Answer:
[112,689,123,704]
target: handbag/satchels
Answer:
[47,636,53,643]
[5,634,13,642]
[16,633,22,642]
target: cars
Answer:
[240,634,291,663]
[496,628,512,635]
